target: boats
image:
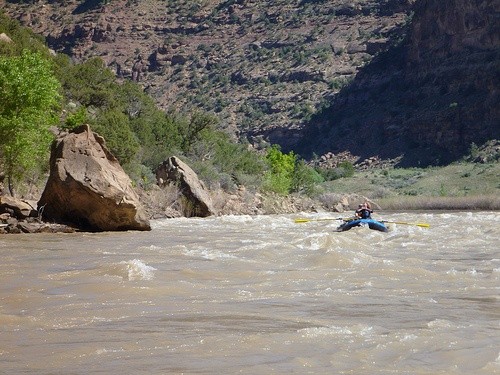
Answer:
[341,215,388,233]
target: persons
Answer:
[355,202,373,220]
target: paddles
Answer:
[293,218,431,229]
